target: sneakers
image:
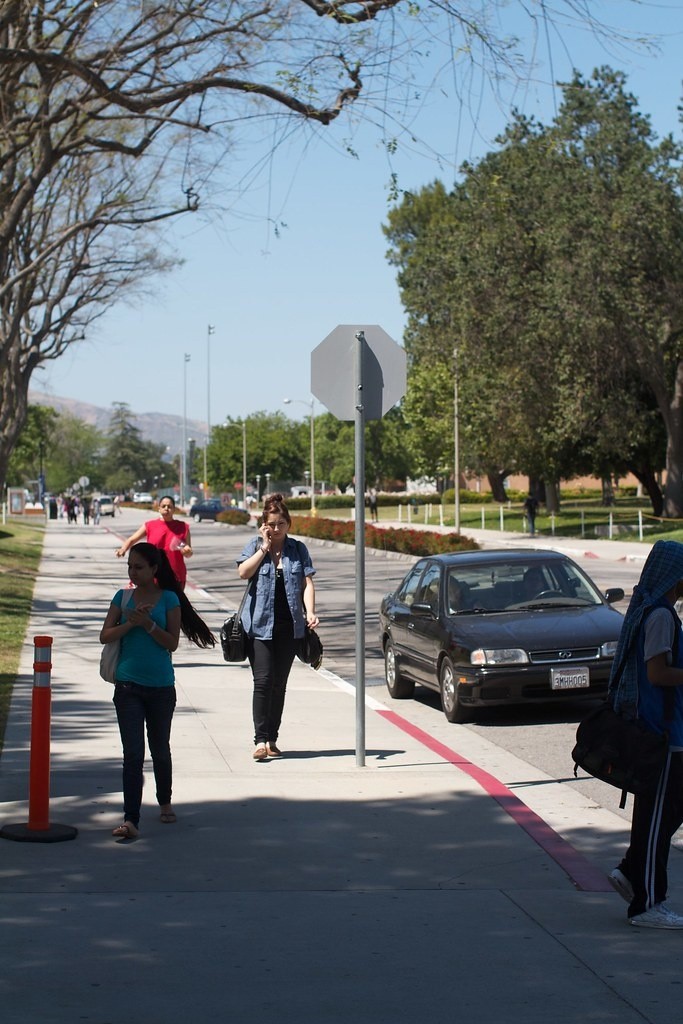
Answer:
[608,869,634,903]
[629,902,683,930]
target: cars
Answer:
[379,548,626,724]
[189,499,251,523]
[132,492,152,503]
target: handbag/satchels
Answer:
[296,623,324,669]
[99,589,133,683]
[572,705,669,798]
[220,613,249,662]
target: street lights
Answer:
[256,475,261,509]
[283,399,316,517]
[166,446,183,507]
[207,325,216,442]
[265,474,271,494]
[182,353,191,501]
[39,440,45,504]
[304,471,311,496]
[223,423,246,510]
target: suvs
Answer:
[91,496,114,518]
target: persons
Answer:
[114,496,193,592]
[100,542,219,838]
[55,495,101,526]
[571,540,683,929]
[523,568,543,602]
[220,494,324,759]
[523,492,540,537]
[436,575,487,612]
[367,488,379,523]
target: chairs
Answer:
[426,576,465,611]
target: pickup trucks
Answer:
[291,480,342,496]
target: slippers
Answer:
[160,811,177,823]
[111,826,137,838]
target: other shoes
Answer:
[253,748,268,759]
[266,746,283,758]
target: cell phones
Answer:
[264,527,272,540]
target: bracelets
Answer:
[147,622,156,634]
